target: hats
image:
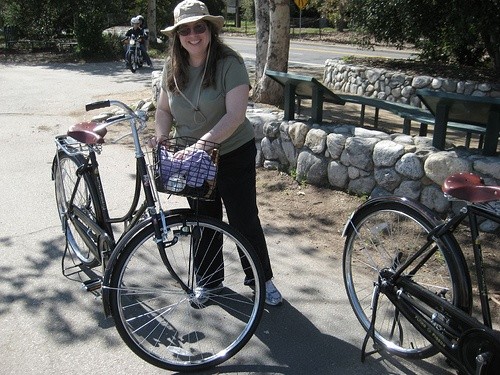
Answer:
[160,0,224,37]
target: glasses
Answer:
[177,23,207,35]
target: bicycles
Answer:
[50,99,267,374]
[340,172,500,375]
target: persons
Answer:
[125,15,154,69]
[147,0,283,306]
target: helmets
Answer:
[131,18,140,26]
[136,15,144,24]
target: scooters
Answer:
[123,34,148,73]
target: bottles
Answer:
[164,173,187,192]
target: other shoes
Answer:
[150,64,153,69]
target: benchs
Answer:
[336,94,487,149]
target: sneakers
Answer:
[250,280,282,305]
[189,283,223,305]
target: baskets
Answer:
[152,136,220,201]
